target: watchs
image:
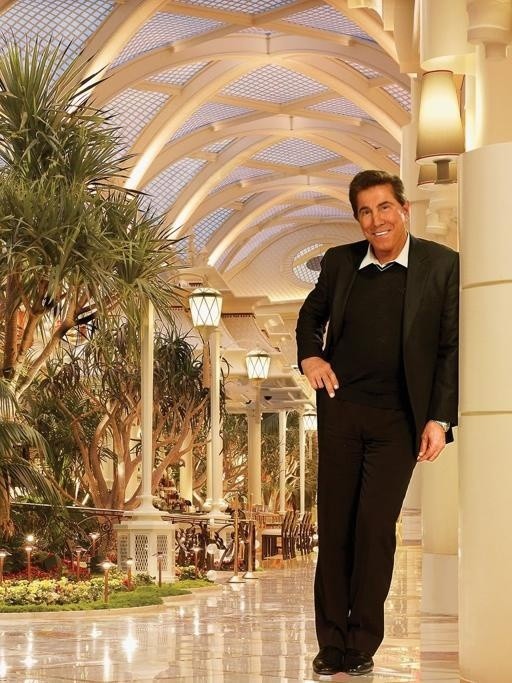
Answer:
[436,419,451,433]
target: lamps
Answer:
[244,343,274,424]
[188,283,226,388]
[408,63,467,185]
[302,403,317,459]
[0,528,204,606]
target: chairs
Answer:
[262,507,313,561]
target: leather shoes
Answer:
[343,649,374,675]
[313,646,344,675]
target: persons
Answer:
[296,168,458,676]
